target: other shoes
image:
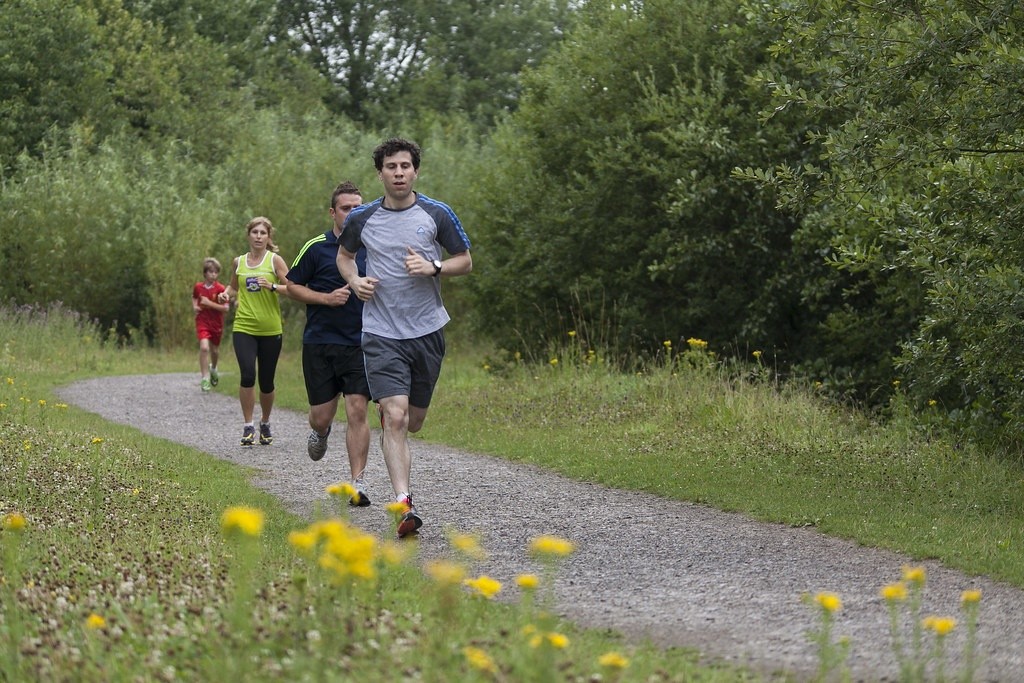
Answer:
[202,378,210,392]
[208,363,218,386]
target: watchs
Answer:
[270,284,276,292]
[431,260,441,277]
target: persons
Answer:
[218,216,289,445]
[284,182,372,507]
[337,137,472,538]
[192,258,230,391]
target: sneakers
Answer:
[241,425,255,445]
[259,422,273,445]
[395,494,423,538]
[308,424,331,461]
[350,485,370,506]
